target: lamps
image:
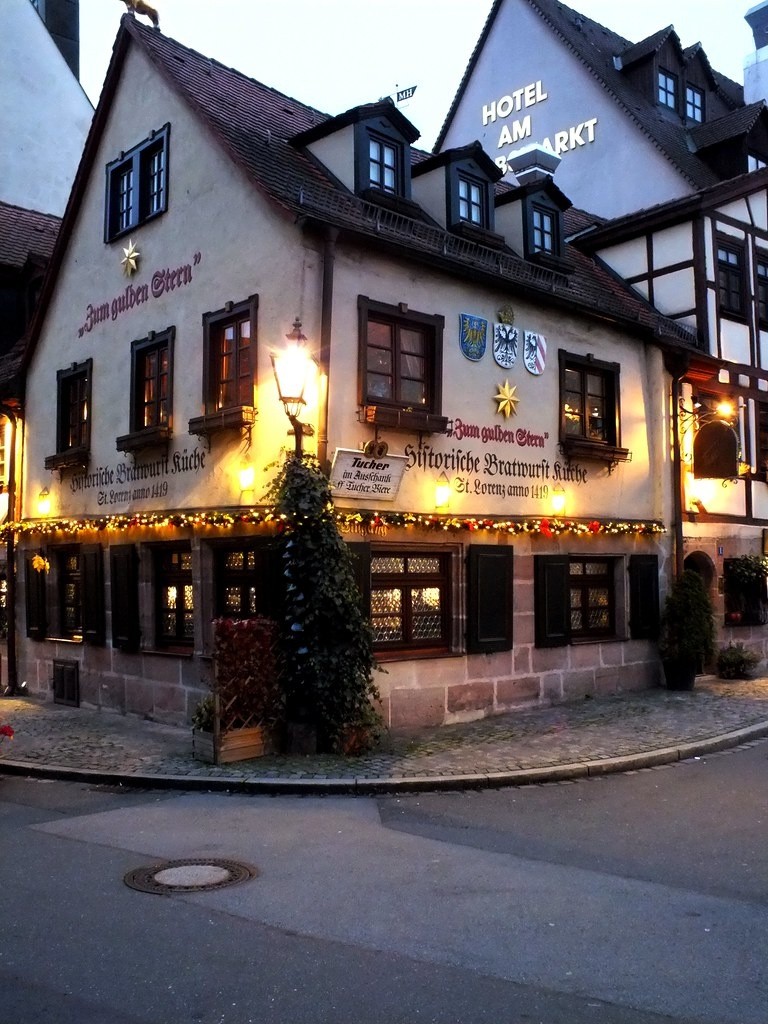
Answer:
[239,452,255,494]
[38,486,50,515]
[270,316,320,446]
[434,471,451,507]
[552,484,566,510]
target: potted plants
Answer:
[257,447,377,754]
[717,641,764,681]
[654,567,716,691]
[193,614,273,765]
